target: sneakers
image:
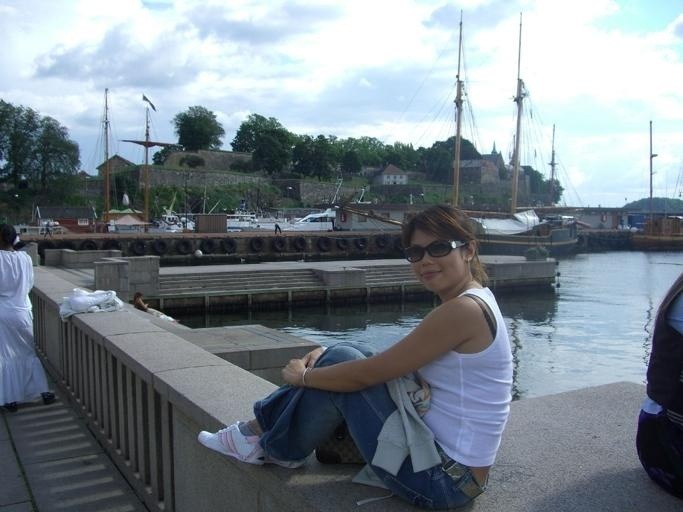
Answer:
[197,421,266,465]
[264,449,307,468]
[0,401,18,412]
[42,392,55,404]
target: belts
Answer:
[435,443,486,500]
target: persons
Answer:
[274,215,282,235]
[635,274,683,499]
[44,222,52,237]
[0,223,56,413]
[197,202,514,512]
[133,291,164,319]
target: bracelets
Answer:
[302,367,309,386]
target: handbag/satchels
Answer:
[316,417,367,466]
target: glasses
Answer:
[401,239,466,263]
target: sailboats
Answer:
[616,118,683,250]
